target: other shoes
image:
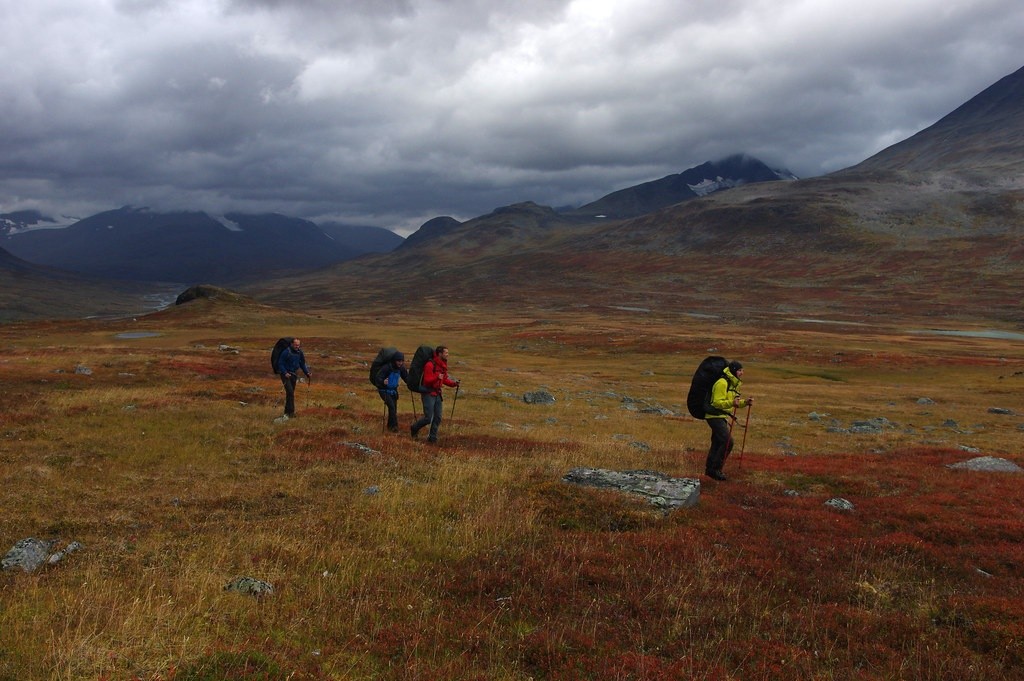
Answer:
[410,425,419,440]
[289,412,297,418]
[705,468,728,481]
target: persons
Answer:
[376,351,409,433]
[411,346,460,442]
[277,337,313,418]
[704,360,753,482]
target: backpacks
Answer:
[369,347,399,388]
[407,344,437,394]
[271,336,295,375]
[687,356,732,420]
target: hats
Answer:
[393,351,404,361]
[729,360,743,374]
